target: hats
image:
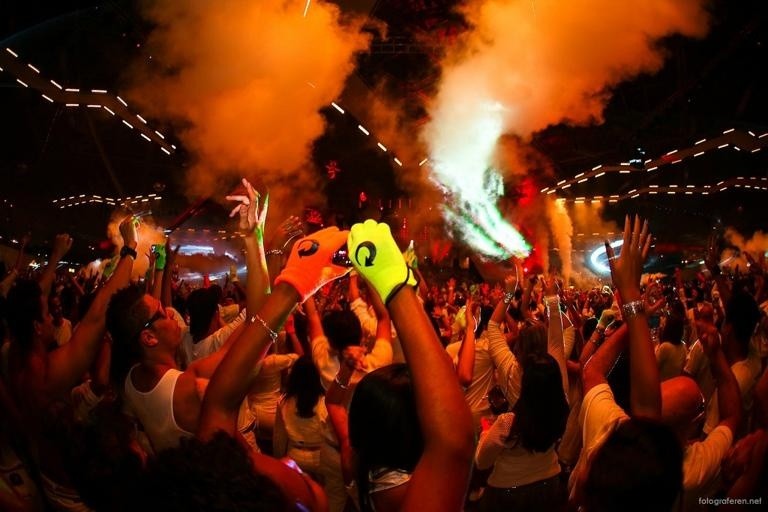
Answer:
[185,285,221,322]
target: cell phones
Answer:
[151,245,156,253]
[332,245,354,267]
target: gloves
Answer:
[155,244,166,270]
[272,225,354,305]
[346,219,420,306]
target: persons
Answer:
[0,179,768,512]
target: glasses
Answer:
[139,300,167,331]
